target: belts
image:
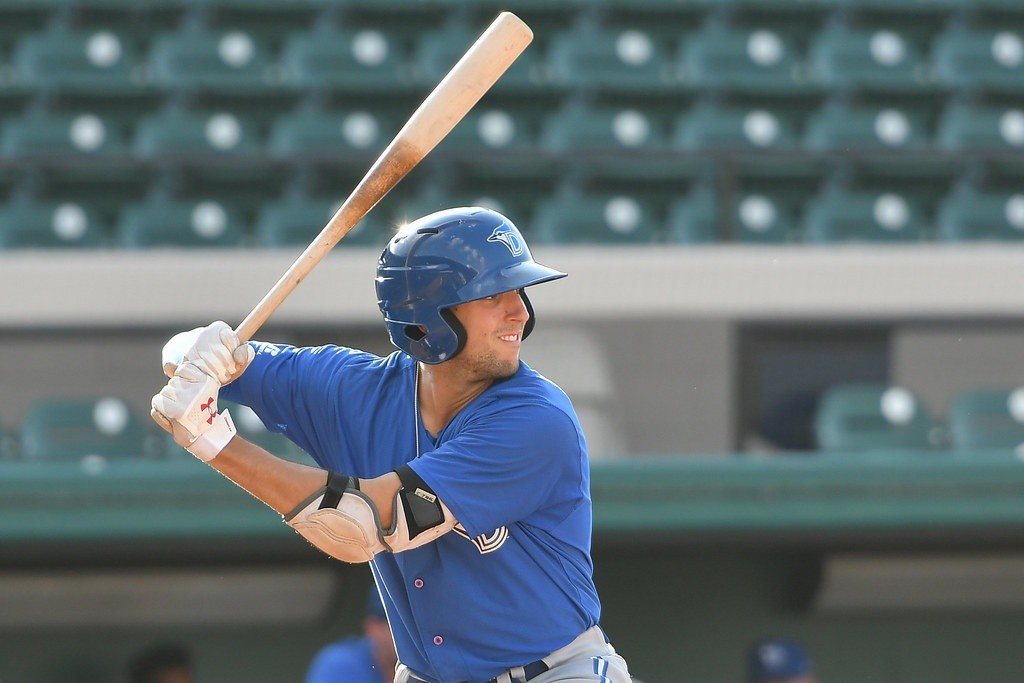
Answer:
[405,625,609,683]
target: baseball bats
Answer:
[152,11,534,423]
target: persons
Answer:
[150,206,638,683]
[134,577,815,683]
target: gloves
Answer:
[151,364,236,462]
[180,321,256,388]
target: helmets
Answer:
[375,207,567,364]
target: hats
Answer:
[752,644,807,678]
